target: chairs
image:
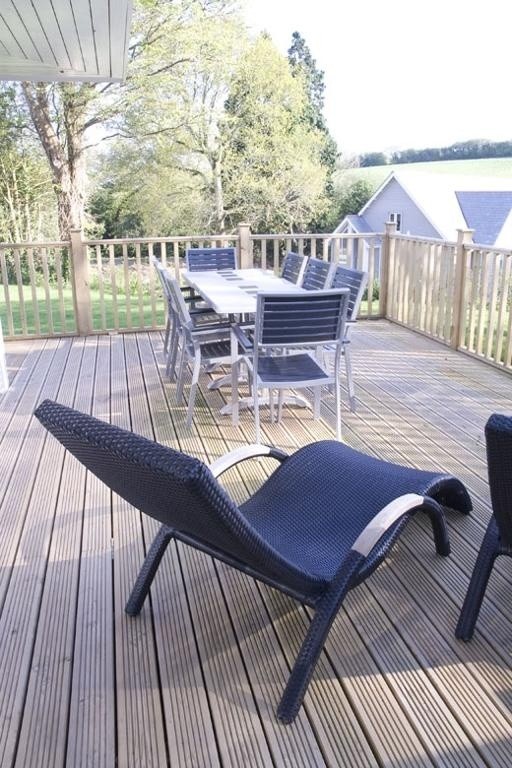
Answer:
[451,412,512,645]
[152,247,369,443]
[30,397,474,724]
